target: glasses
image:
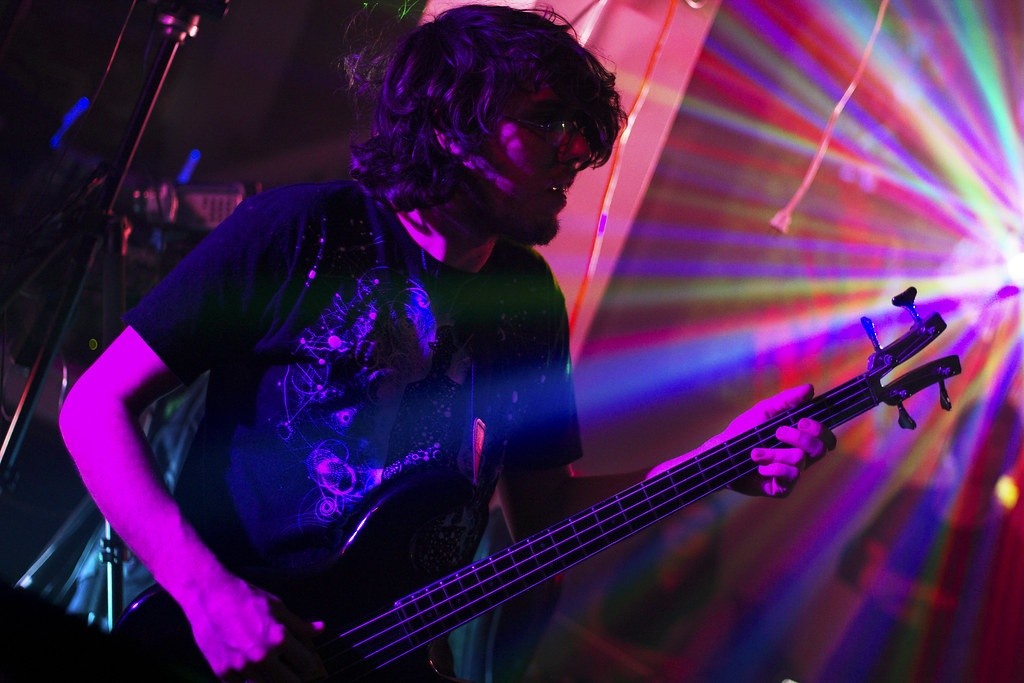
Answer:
[484,108,601,171]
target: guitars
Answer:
[101,286,962,682]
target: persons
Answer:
[575,398,1024,683]
[57,5,837,683]
